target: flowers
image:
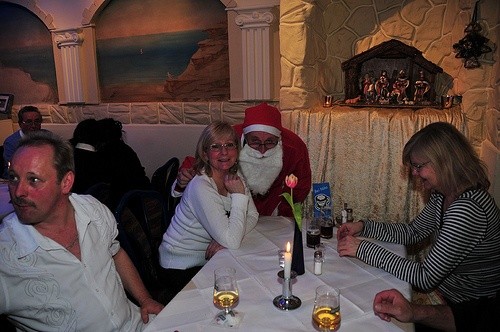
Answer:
[279,173,302,231]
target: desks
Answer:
[144,214,412,332]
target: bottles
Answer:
[278,249,286,268]
[313,251,322,275]
[336,203,354,226]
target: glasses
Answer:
[245,138,278,150]
[205,143,239,151]
[409,161,430,170]
[23,120,41,126]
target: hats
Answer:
[242,103,282,137]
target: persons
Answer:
[159,120,260,293]
[64,118,151,233]
[0,134,165,332]
[3,106,54,167]
[338,122,500,303]
[374,288,500,332]
[187,103,312,218]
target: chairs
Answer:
[152,158,181,192]
[115,189,175,306]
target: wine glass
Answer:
[212,267,240,324]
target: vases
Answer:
[292,218,305,275]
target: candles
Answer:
[284,252,291,278]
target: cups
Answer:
[322,95,334,107]
[318,214,333,239]
[441,94,452,108]
[306,218,321,248]
[311,285,341,332]
